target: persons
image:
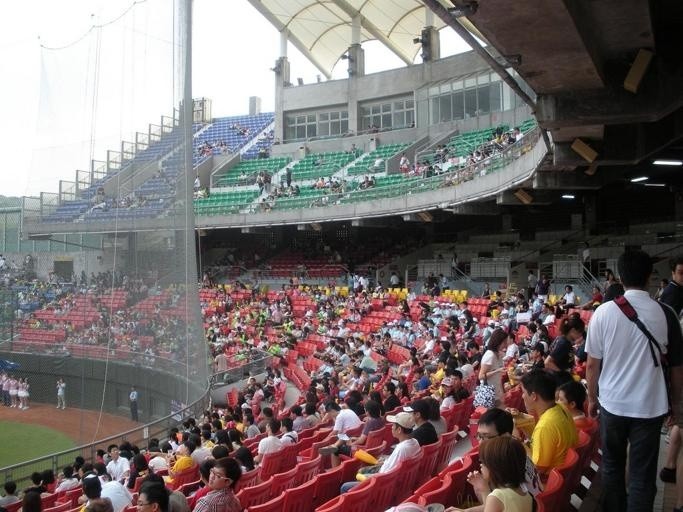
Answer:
[350,143,357,153]
[156,170,177,202]
[241,372,400,494]
[238,167,300,213]
[196,138,227,157]
[0,437,163,512]
[314,176,347,194]
[360,175,376,190]
[402,269,585,369]
[97,183,163,209]
[660,257,683,483]
[491,241,524,251]
[56,377,66,410]
[229,121,248,136]
[367,154,384,173]
[444,126,525,186]
[603,271,616,292]
[256,131,280,160]
[367,123,378,133]
[583,287,602,308]
[163,373,241,512]
[653,279,668,299]
[192,175,209,198]
[0,373,30,411]
[584,248,669,512]
[129,385,139,424]
[400,370,591,512]
[312,152,322,165]
[399,144,448,177]
[451,252,458,269]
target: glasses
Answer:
[137,502,154,507]
[473,433,502,442]
[210,469,231,480]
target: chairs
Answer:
[344,475,377,512]
[391,495,426,506]
[316,495,346,512]
[47,110,541,224]
[234,429,284,455]
[0,246,594,415]
[261,446,288,480]
[283,439,303,474]
[244,476,274,510]
[234,466,262,494]
[414,474,453,508]
[235,490,247,511]
[249,491,287,512]
[440,424,459,470]
[317,461,344,507]
[0,433,234,511]
[298,436,337,463]
[531,496,545,512]
[346,423,364,438]
[440,457,473,505]
[375,463,403,512]
[401,447,424,503]
[573,431,592,486]
[286,476,318,512]
[297,418,335,442]
[365,439,388,466]
[556,447,579,507]
[385,423,394,452]
[273,465,298,498]
[344,452,366,483]
[299,454,322,488]
[536,468,564,512]
[463,446,481,476]
[423,435,444,485]
[351,427,387,458]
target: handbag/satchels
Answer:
[471,373,497,409]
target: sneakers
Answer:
[62,407,67,410]
[662,425,668,434]
[11,405,15,408]
[659,468,677,484]
[57,407,61,409]
[15,405,18,408]
[319,446,339,457]
[665,436,671,446]
[23,407,30,411]
[20,403,23,409]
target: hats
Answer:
[441,378,454,386]
[387,413,416,428]
[403,400,430,413]
